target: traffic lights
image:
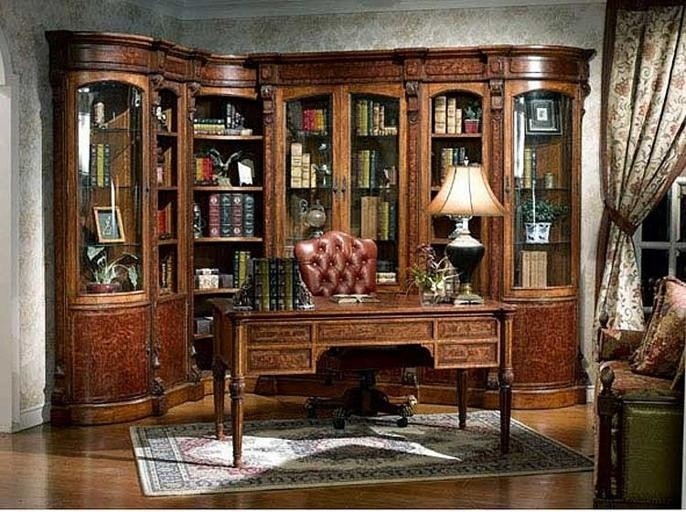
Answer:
[628,276,686,378]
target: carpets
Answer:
[129,411,595,498]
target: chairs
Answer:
[293,229,414,430]
[592,311,686,509]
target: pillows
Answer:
[628,276,686,378]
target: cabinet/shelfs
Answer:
[44,29,598,426]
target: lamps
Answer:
[293,229,414,430]
[592,311,686,509]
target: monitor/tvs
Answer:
[516,249,548,289]
[525,149,532,188]
[328,293,382,304]
[253,259,295,312]
[515,149,525,188]
[82,142,110,188]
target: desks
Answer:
[205,293,517,468]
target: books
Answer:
[355,100,396,137]
[516,249,548,289]
[290,143,317,188]
[160,256,175,287]
[375,260,396,284]
[351,150,377,189]
[233,251,253,286]
[194,156,214,186]
[359,196,397,241]
[227,104,244,129]
[434,99,462,134]
[253,259,295,312]
[193,118,225,135]
[328,293,382,304]
[208,194,253,237]
[82,142,110,188]
[288,102,328,131]
[525,149,532,188]
[440,147,465,186]
[515,149,525,188]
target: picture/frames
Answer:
[205,293,517,468]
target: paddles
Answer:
[129,411,595,498]
